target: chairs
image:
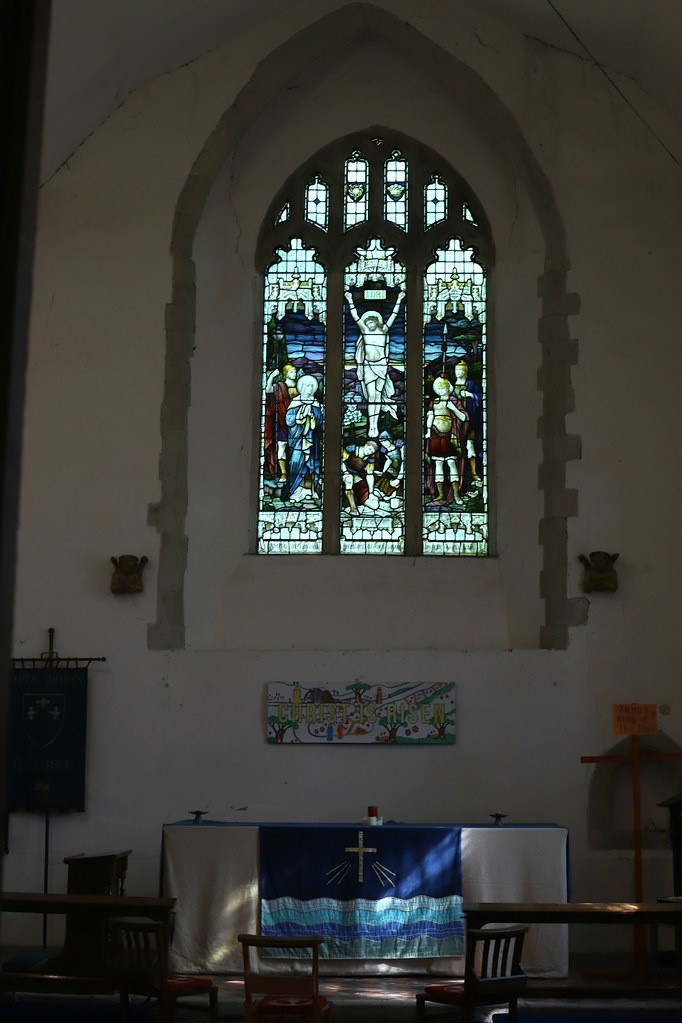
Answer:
[416,924,529,1023]
[110,922,219,1023]
[238,934,332,1023]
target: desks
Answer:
[160,819,569,977]
